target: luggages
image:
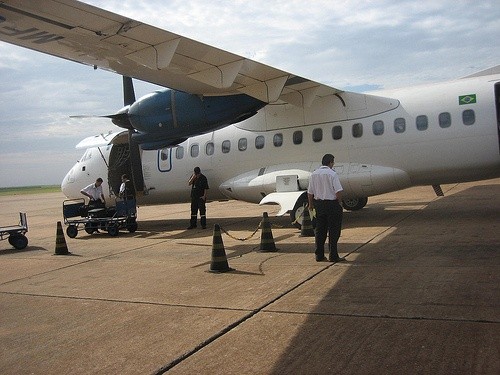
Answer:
[116,201,126,217]
[87,208,106,218]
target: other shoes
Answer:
[329,257,346,261]
[187,224,197,229]
[94,230,100,233]
[317,257,329,262]
[202,225,206,229]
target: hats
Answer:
[194,167,200,173]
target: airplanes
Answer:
[0,0,500,230]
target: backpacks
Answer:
[106,204,117,217]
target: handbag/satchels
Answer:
[79,205,97,217]
[89,197,102,205]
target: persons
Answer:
[81,178,109,234]
[186,166,209,229]
[308,154,346,263]
[119,174,138,233]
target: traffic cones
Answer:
[204,224,238,273]
[298,206,316,237]
[256,211,280,253]
[50,220,74,256]
